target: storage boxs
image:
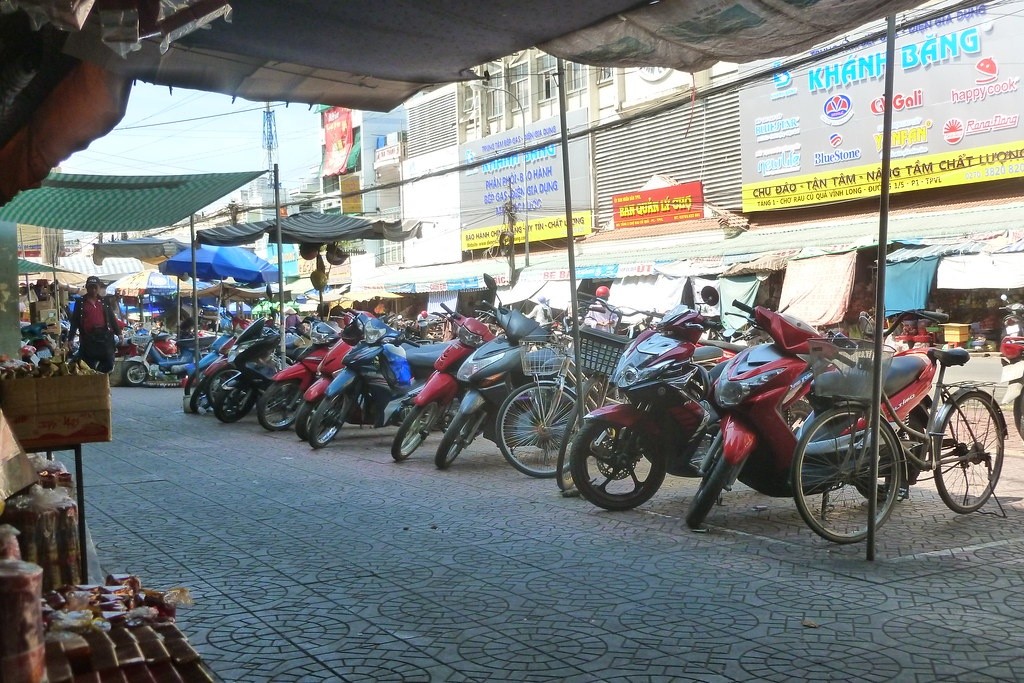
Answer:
[29,301,57,323]
[944,326,968,342]
[0,373,112,448]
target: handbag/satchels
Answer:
[83,332,114,359]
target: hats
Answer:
[87,276,101,286]
[285,308,297,314]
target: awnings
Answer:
[279,229,1024,328]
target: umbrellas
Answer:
[17,209,435,324]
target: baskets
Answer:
[807,338,896,403]
[131,333,151,346]
[519,335,566,376]
[578,323,635,375]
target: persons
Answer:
[523,297,553,325]
[584,287,618,334]
[417,310,434,322]
[38,276,125,377]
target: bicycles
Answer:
[790,308,1010,544]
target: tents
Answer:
[2,164,287,385]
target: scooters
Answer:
[684,297,938,531]
[12,271,1024,513]
